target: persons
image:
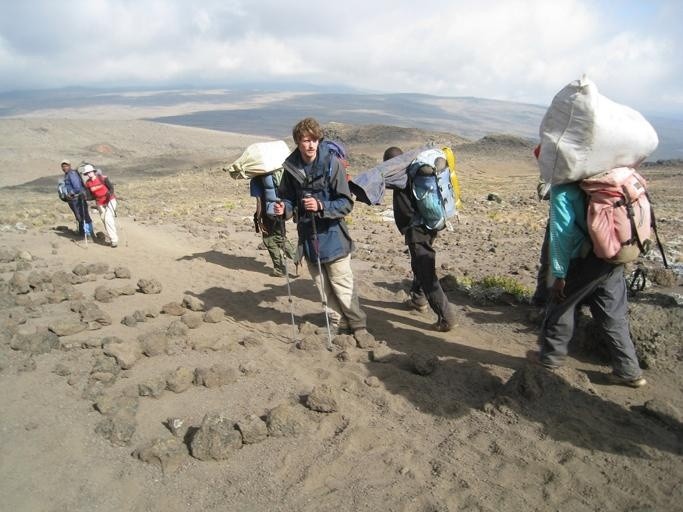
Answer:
[272,116,369,336]
[57,159,95,238]
[80,164,117,247]
[524,145,558,329]
[381,145,459,332]
[541,173,649,387]
[245,167,302,276]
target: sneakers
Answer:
[599,368,647,387]
[353,328,376,348]
[315,324,350,335]
[431,322,459,332]
[526,350,557,370]
[404,296,429,314]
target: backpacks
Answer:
[401,148,459,232]
[262,169,284,223]
[577,166,651,266]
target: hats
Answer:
[61,160,71,166]
[83,165,97,175]
